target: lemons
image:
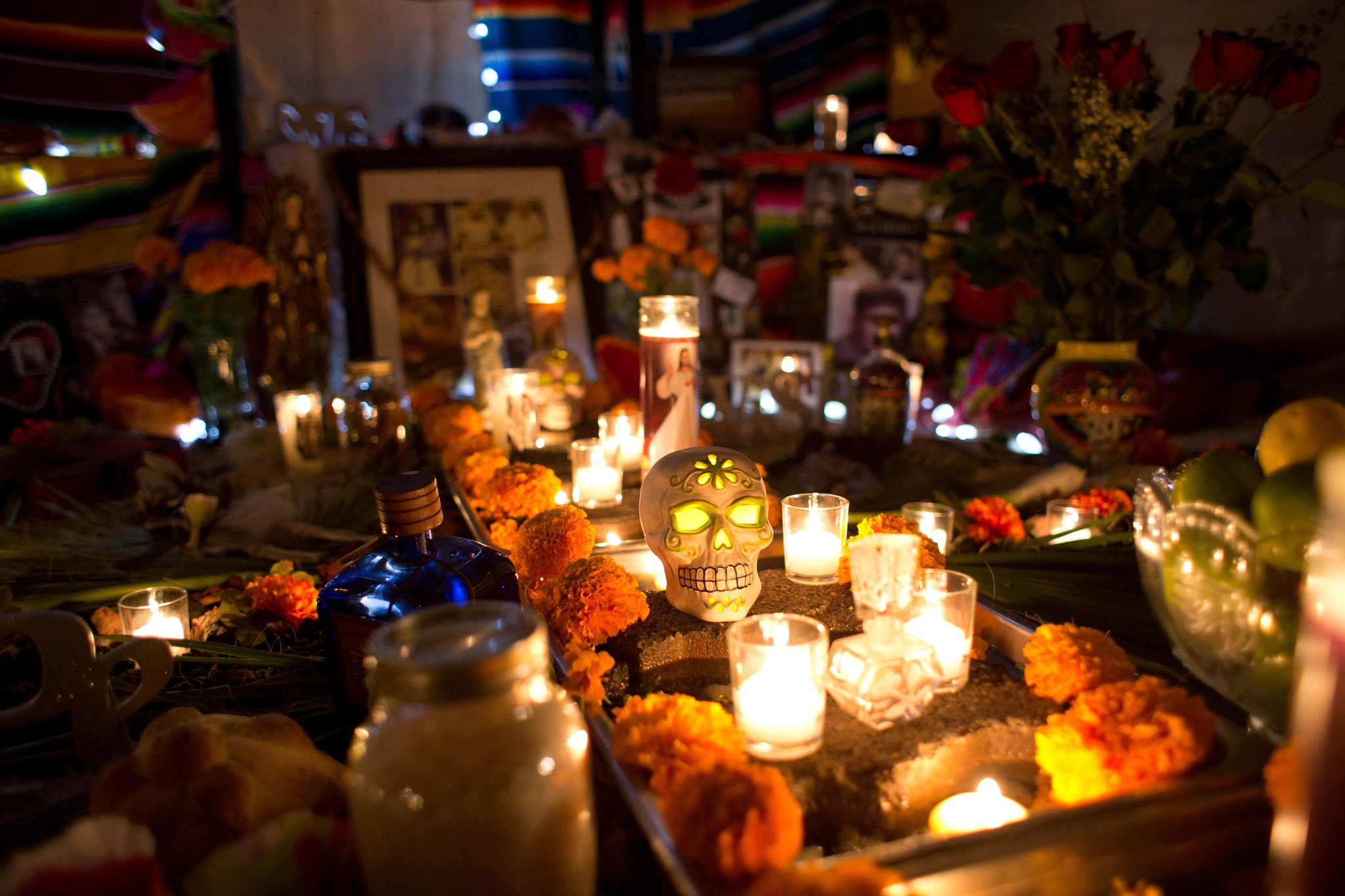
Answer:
[1164,398,1345,727]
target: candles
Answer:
[133,600,183,641]
[905,600,965,676]
[787,511,840,574]
[737,621,821,742]
[574,448,620,500]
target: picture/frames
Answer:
[325,132,609,393]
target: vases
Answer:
[1032,335,1156,470]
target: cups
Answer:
[484,368,543,451]
[118,586,191,656]
[571,438,624,509]
[1044,499,1099,544]
[726,613,829,760]
[894,568,978,694]
[781,493,850,585]
[597,410,644,471]
[274,389,324,476]
[901,502,954,556]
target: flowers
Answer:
[93,27,1326,896]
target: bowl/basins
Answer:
[1132,456,1312,747]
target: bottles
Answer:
[347,601,597,896]
[1030,340,1158,472]
[322,358,418,461]
[316,471,520,722]
